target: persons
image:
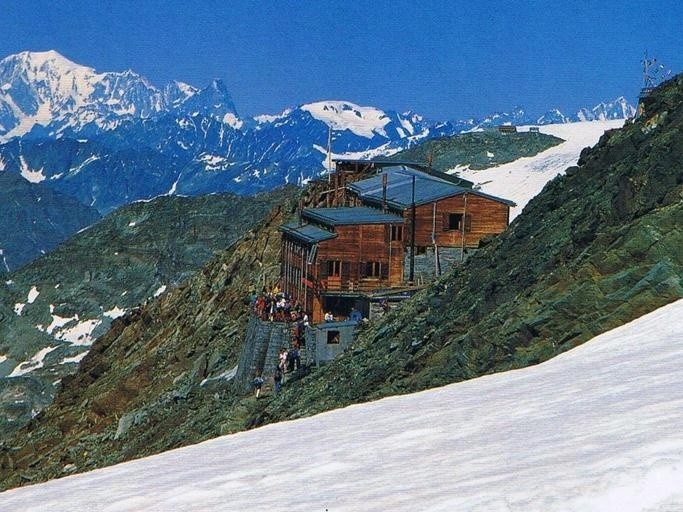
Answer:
[324,310,334,322]
[254,283,311,398]
[349,306,362,321]
[379,296,391,315]
